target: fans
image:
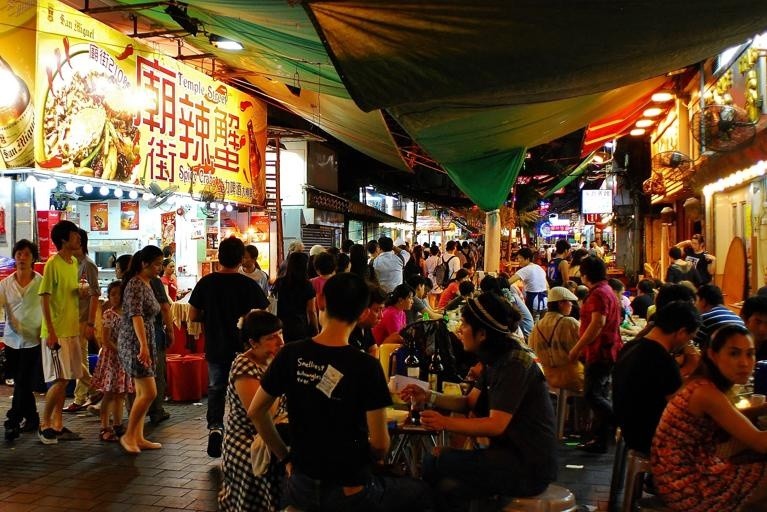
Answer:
[651,145,702,181]
[691,98,762,153]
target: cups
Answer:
[750,394,766,407]
[411,409,424,425]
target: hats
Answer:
[288,241,305,252]
[309,245,328,256]
[547,286,579,303]
[466,292,522,334]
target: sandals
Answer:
[139,440,162,450]
[114,423,127,437]
[99,427,120,443]
[120,435,140,453]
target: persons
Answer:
[616,285,697,363]
[126,277,174,428]
[268,252,319,342]
[528,286,613,432]
[562,281,580,320]
[616,300,702,512]
[61,228,100,412]
[159,259,189,303]
[397,293,557,512]
[496,277,534,345]
[650,322,766,512]
[188,236,272,458]
[631,278,655,319]
[37,219,91,445]
[446,282,476,310]
[693,284,750,353]
[572,285,591,318]
[217,310,290,511]
[159,246,175,277]
[116,244,164,455]
[246,273,433,512]
[608,278,626,325]
[506,247,549,324]
[418,278,449,318]
[0,239,44,440]
[684,233,717,286]
[439,269,469,311]
[349,289,387,359]
[480,275,526,345]
[87,254,133,414]
[741,295,767,393]
[527,238,614,277]
[665,246,702,286]
[407,274,463,325]
[498,271,525,302]
[370,284,416,346]
[88,281,127,442]
[569,255,624,451]
[280,236,520,282]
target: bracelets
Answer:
[429,390,437,404]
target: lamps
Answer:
[211,40,244,50]
[23,176,234,213]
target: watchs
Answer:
[443,308,447,316]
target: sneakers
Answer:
[63,402,85,413]
[37,427,58,444]
[58,428,79,441]
[81,388,103,410]
[207,424,224,458]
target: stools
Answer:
[501,484,576,512]
[549,385,592,440]
[608,428,626,511]
[622,450,650,512]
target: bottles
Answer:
[443,310,449,323]
[404,342,420,380]
[428,349,443,393]
[621,314,629,330]
[423,313,429,321]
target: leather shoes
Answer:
[5,425,20,440]
[20,413,40,432]
[576,439,607,455]
[150,413,170,428]
